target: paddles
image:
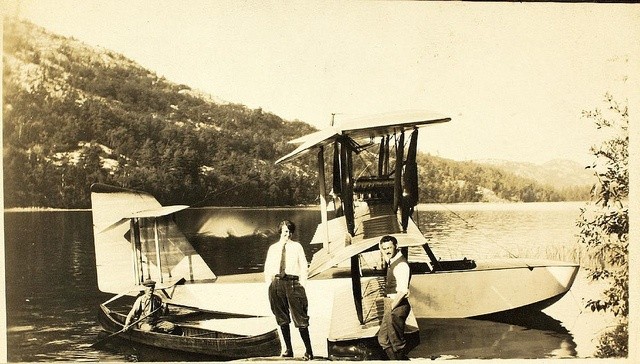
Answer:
[88,305,163,350]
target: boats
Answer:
[99,293,282,359]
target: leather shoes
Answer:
[304,351,313,360]
[282,348,293,357]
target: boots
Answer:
[394,345,409,360]
[384,346,398,361]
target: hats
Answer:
[142,280,156,287]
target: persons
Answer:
[263,220,314,361]
[123,280,177,335]
[376,236,412,359]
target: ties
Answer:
[387,258,392,268]
[279,243,286,278]
[149,297,154,314]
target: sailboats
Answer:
[91,113,579,318]
